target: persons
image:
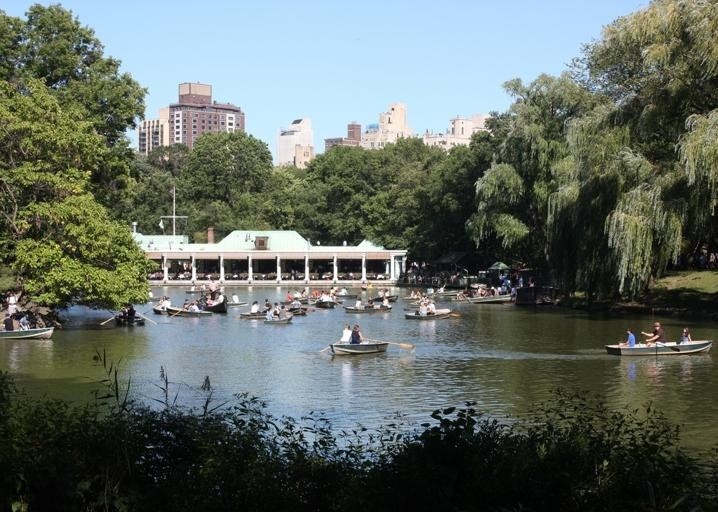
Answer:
[682,328,692,344]
[619,328,635,348]
[335,324,364,345]
[0,311,46,331]
[641,322,666,347]
[118,305,136,320]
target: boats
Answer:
[604,340,713,355]
[329,341,389,354]
[0,326,54,339]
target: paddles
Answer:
[654,342,680,352]
[435,312,462,318]
[363,339,415,349]
